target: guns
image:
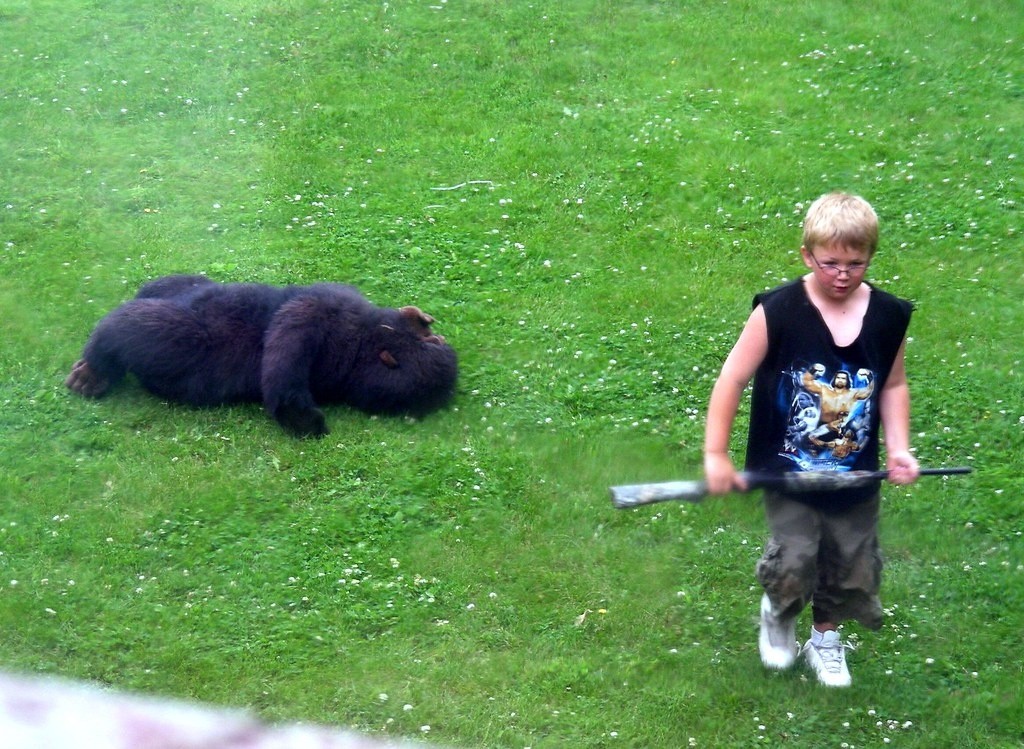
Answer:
[609,464,976,512]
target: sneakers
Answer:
[801,629,852,687]
[759,591,798,671]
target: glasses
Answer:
[804,240,870,279]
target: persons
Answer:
[703,193,919,687]
